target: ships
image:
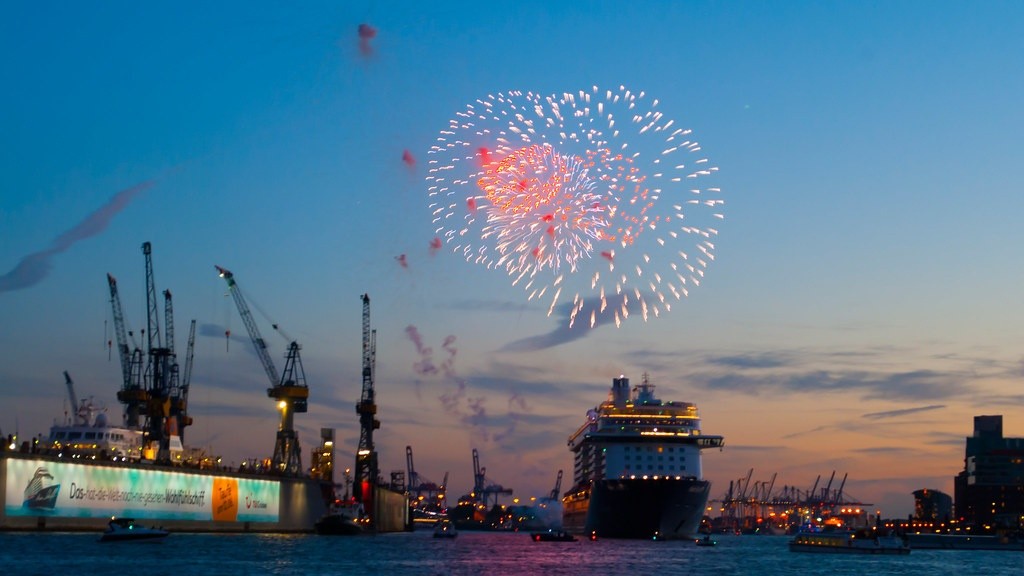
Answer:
[20,467,60,509]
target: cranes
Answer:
[352,294,380,509]
[215,264,310,479]
[105,240,197,465]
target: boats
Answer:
[432,521,457,541]
[786,523,910,554]
[696,538,717,547]
[562,373,724,539]
[33,368,564,533]
[531,529,575,541]
[315,499,370,536]
[94,519,174,545]
